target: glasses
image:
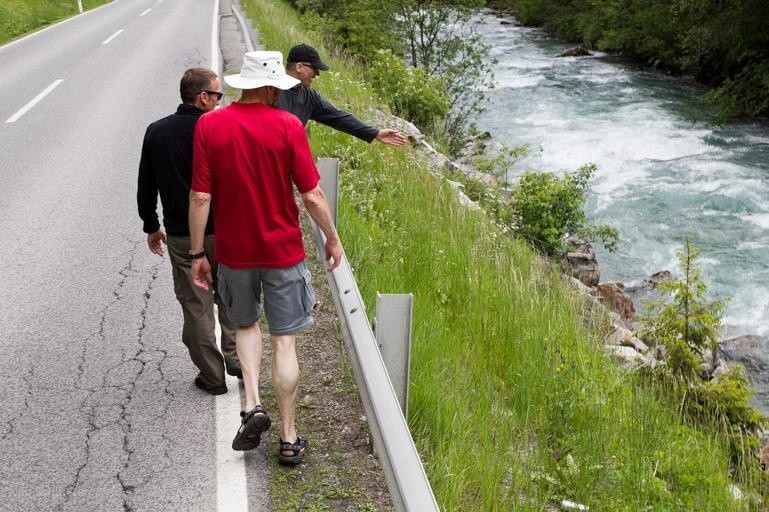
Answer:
[193,89,223,100]
[302,63,320,76]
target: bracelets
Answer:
[186,248,206,261]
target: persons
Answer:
[270,43,407,151]
[135,68,243,395]
[187,50,344,467]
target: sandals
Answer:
[232,405,271,451]
[278,436,308,464]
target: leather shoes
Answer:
[194,375,227,395]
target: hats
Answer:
[287,44,329,70]
[224,50,302,90]
